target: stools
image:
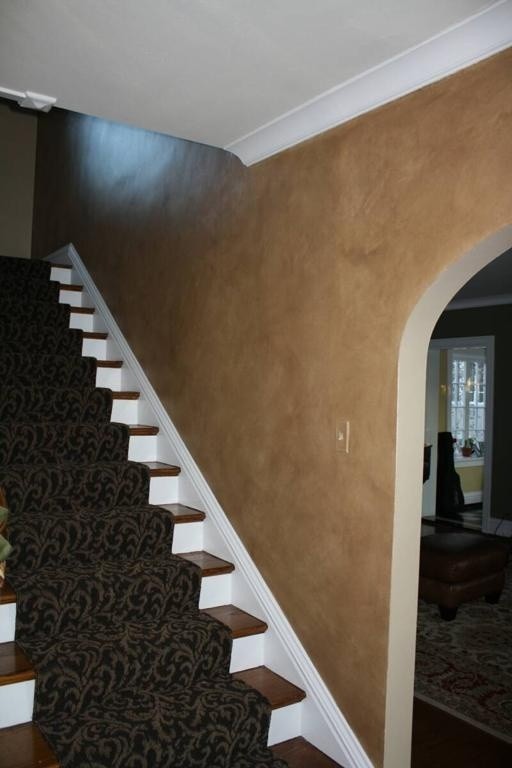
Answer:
[418,532,510,622]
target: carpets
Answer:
[413,588,511,746]
[0,255,285,765]
